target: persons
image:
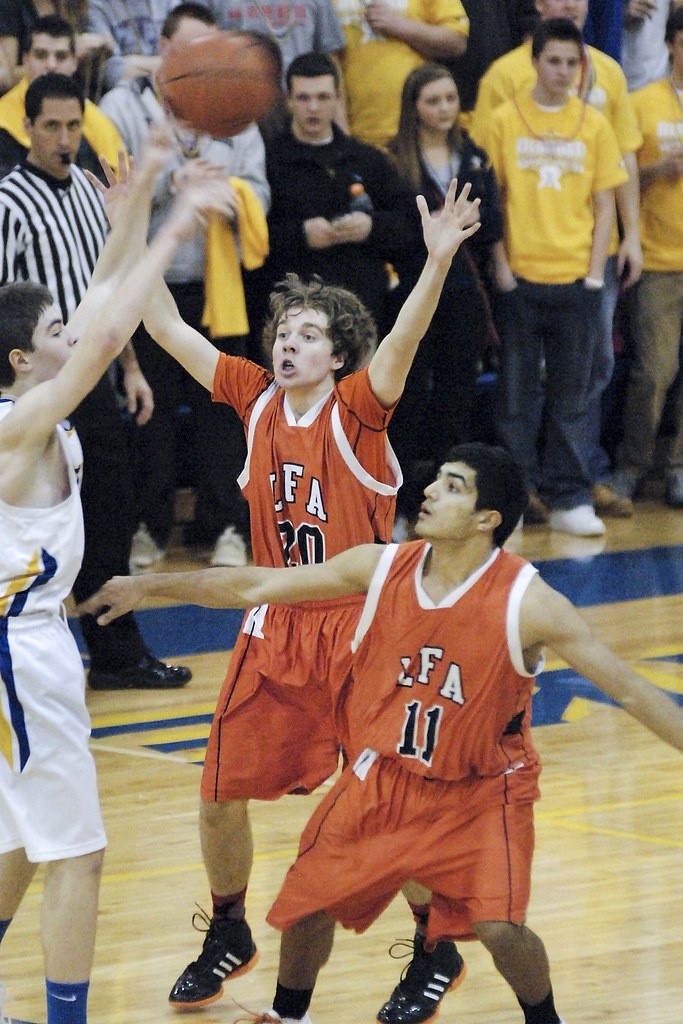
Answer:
[0,0,683,1024]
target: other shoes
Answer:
[524,491,548,522]
[610,471,637,499]
[591,483,634,516]
[132,522,166,567]
[550,506,606,536]
[664,475,683,507]
[210,526,247,567]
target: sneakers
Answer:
[87,650,192,690]
[253,1005,311,1024]
[375,932,467,1024]
[168,901,260,1008]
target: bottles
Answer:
[349,182,375,214]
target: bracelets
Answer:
[583,277,604,288]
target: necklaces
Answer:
[421,141,454,192]
[513,95,586,142]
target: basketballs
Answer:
[155,30,277,140]
